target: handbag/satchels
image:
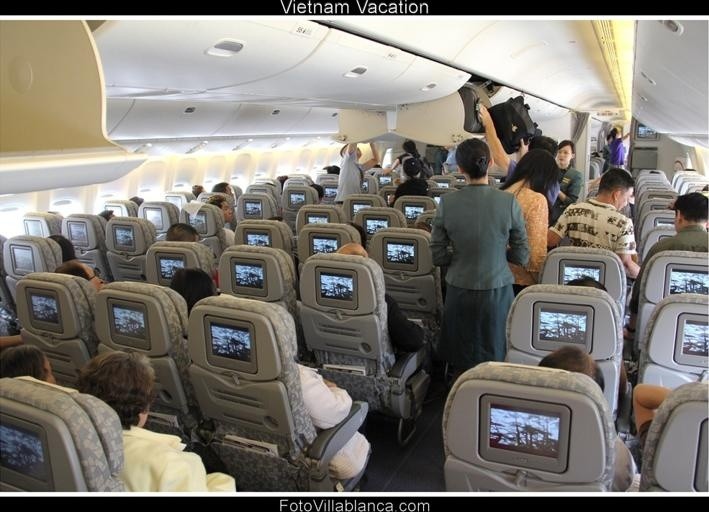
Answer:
[487,95,538,154]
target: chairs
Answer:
[2,170,707,512]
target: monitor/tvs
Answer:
[0,174,709,373]
[635,121,660,140]
[0,411,54,492]
[477,394,571,474]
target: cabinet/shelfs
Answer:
[1,13,569,196]
[633,19,708,148]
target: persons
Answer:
[206,195,233,229]
[535,347,604,393]
[310,184,324,202]
[211,182,232,196]
[630,193,709,311]
[632,384,672,452]
[47,235,75,262]
[333,143,361,205]
[543,167,641,280]
[0,344,55,385]
[387,158,425,208]
[165,222,198,243]
[71,349,236,494]
[289,360,370,480]
[191,184,204,197]
[382,140,422,177]
[53,260,103,291]
[423,104,630,379]
[338,244,423,354]
[168,267,215,312]
[98,210,114,221]
[130,196,143,205]
[564,276,605,292]
